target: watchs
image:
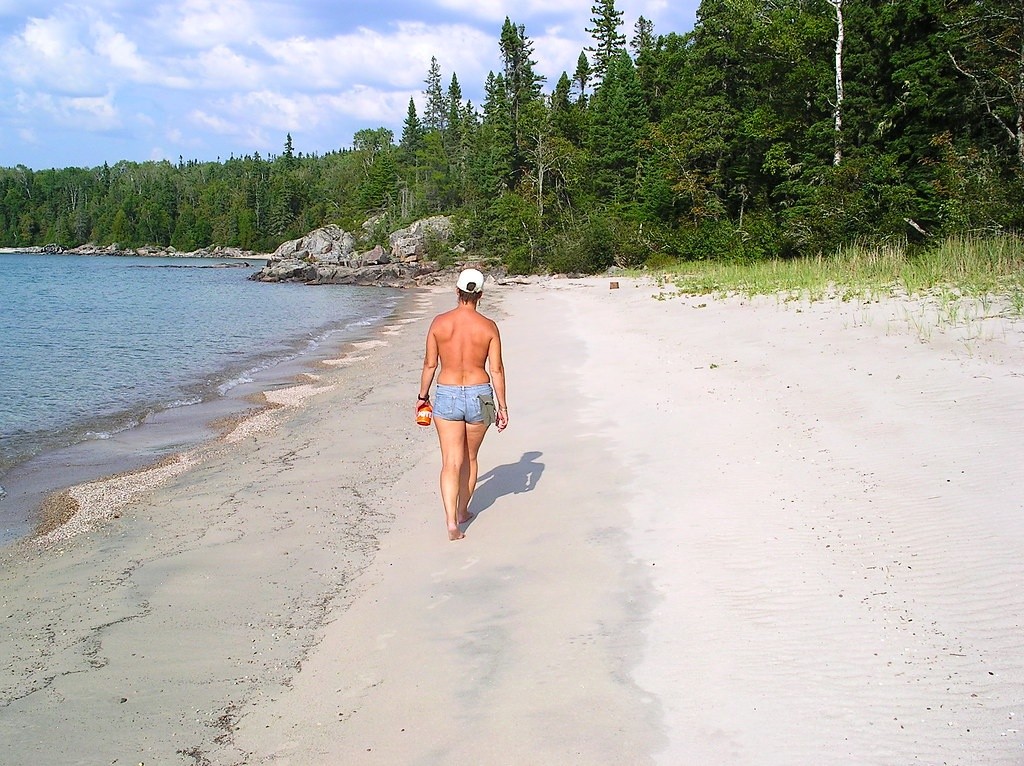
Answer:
[417,394,429,401]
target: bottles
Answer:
[416,400,432,425]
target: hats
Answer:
[457,268,484,293]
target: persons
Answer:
[415,268,509,540]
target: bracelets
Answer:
[499,407,507,411]
